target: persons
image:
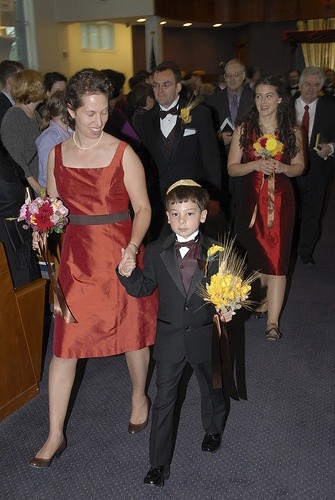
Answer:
[28,68,158,469]
[114,179,260,486]
[0,57,335,342]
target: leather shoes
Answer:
[201,432,221,453]
[143,466,171,488]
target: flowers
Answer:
[6,187,69,234]
[179,94,202,129]
[191,230,263,314]
[253,133,284,160]
[204,244,224,278]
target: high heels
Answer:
[254,300,269,320]
[129,395,151,435]
[265,323,281,344]
[28,434,66,469]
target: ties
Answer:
[301,105,311,147]
[230,95,241,125]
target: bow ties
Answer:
[289,85,300,92]
[158,106,178,121]
[173,239,196,252]
[325,85,333,90]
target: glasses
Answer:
[152,80,174,93]
[220,72,242,82]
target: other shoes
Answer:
[303,254,314,268]
